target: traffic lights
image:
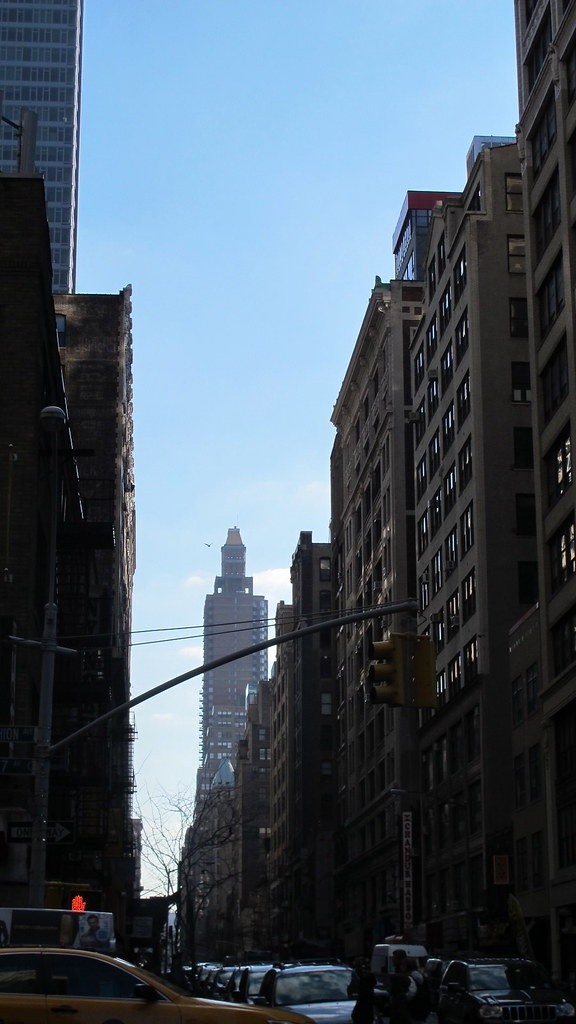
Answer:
[65,889,104,912]
[369,632,400,707]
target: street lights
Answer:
[27,405,69,914]
[387,785,473,956]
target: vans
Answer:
[372,935,429,976]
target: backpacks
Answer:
[408,971,437,1021]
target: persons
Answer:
[80,915,111,948]
[337,937,450,1024]
[266,935,311,964]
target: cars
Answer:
[178,960,293,1005]
[0,907,317,1024]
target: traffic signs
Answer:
[6,819,74,845]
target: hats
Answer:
[96,928,110,942]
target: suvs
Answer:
[439,956,576,1024]
[261,959,385,1024]
